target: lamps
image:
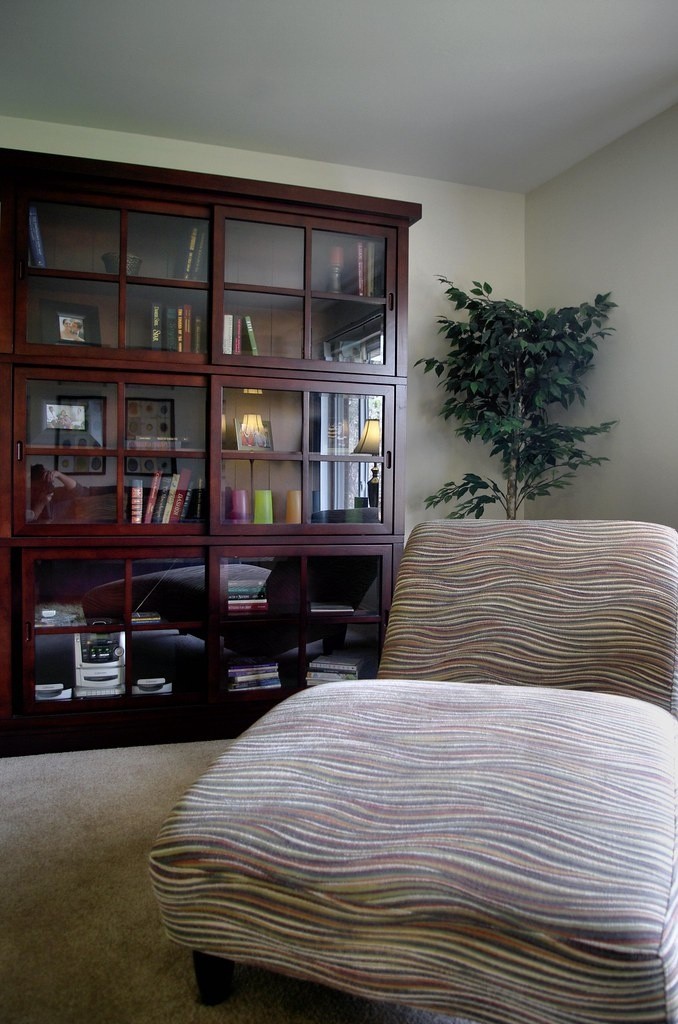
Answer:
[235,414,267,520]
[352,419,381,508]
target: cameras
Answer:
[45,480,55,494]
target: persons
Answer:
[240,425,269,448]
[47,406,84,429]
[26,464,90,524]
[61,319,84,341]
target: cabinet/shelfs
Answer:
[0,149,422,758]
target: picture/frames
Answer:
[41,299,102,346]
[124,397,177,476]
[54,395,107,476]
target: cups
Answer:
[254,489,273,524]
[311,490,319,512]
[231,489,250,524]
[286,489,301,524]
[354,497,369,508]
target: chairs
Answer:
[80,507,386,654]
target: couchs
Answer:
[145,522,678,1024]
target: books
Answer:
[145,226,258,356]
[328,239,385,298]
[34,600,87,627]
[29,208,47,267]
[128,466,204,524]
[131,611,160,625]
[225,573,364,692]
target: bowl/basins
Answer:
[100,252,143,275]
